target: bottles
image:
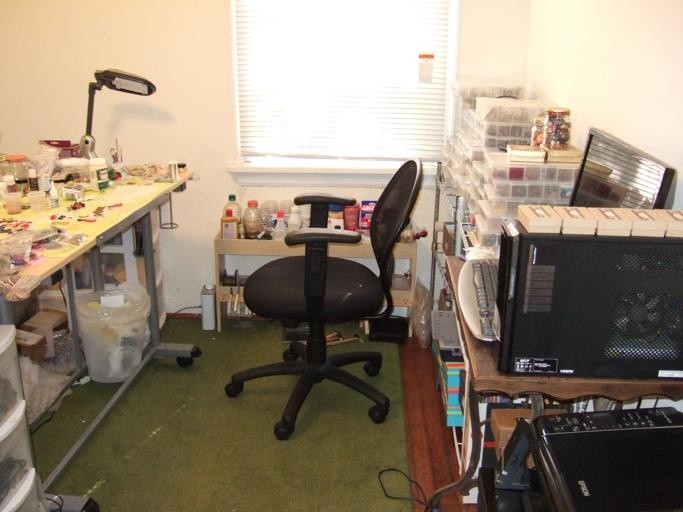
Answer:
[3,154,34,196]
[167,162,178,180]
[4,174,16,192]
[529,117,544,147]
[48,182,59,208]
[27,168,38,190]
[221,194,306,241]
[343,194,360,233]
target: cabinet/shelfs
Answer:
[424,161,477,351]
[212,208,419,340]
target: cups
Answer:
[3,192,21,214]
[26,190,46,213]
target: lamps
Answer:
[78,69,155,160]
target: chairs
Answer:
[224,158,423,440]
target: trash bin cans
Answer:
[74,290,150,383]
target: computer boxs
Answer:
[496,220,683,380]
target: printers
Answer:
[534,406,683,511]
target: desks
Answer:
[0,164,202,511]
[424,254,682,510]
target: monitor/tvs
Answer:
[569,128,673,208]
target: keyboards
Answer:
[457,258,498,342]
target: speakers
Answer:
[493,417,532,489]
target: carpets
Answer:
[28,318,415,512]
